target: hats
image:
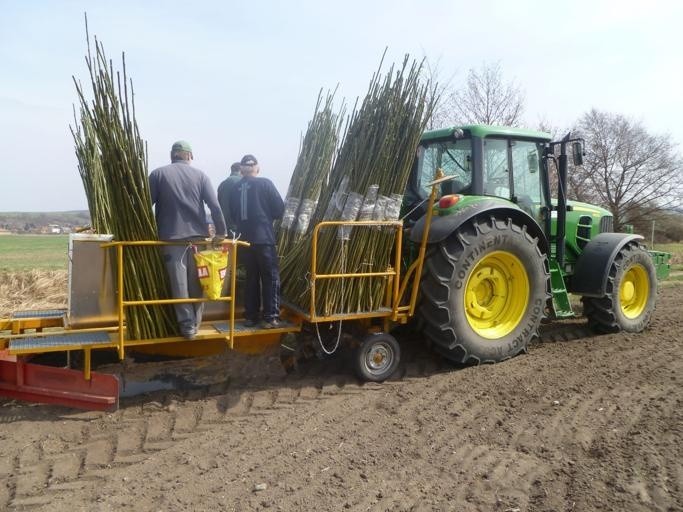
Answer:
[240,155,258,166]
[171,141,192,152]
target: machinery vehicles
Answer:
[1,125,661,414]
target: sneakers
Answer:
[180,325,197,337]
[262,318,280,328]
[245,318,258,326]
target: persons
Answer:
[229,153,287,325]
[147,139,229,341]
[216,160,248,308]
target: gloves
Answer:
[213,236,225,250]
